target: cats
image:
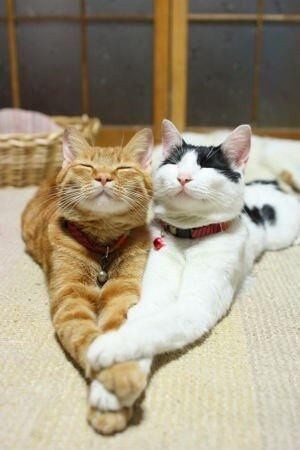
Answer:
[21,126,160,436]
[86,118,299,412]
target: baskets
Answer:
[0,116,101,187]
